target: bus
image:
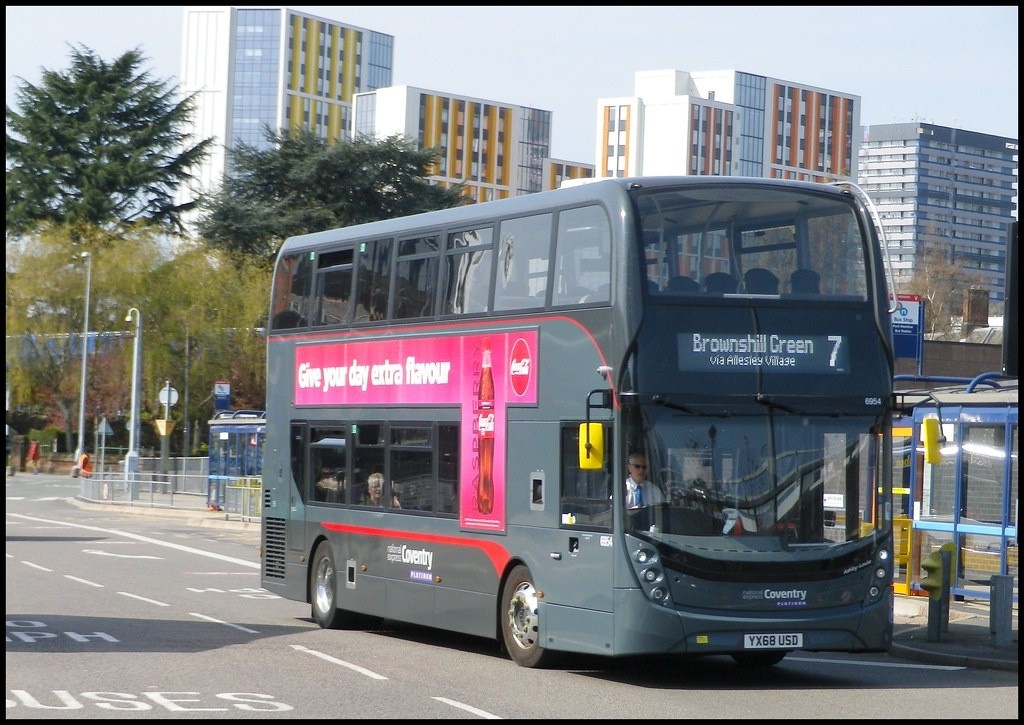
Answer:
[259,171,946,676]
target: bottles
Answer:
[476,338,495,514]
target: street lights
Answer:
[124,308,145,492]
[78,251,92,462]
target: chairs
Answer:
[667,276,701,294]
[744,266,780,295]
[790,268,822,293]
[706,271,738,294]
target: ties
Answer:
[636,486,644,506]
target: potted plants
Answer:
[6,455,16,476]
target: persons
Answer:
[358,472,402,509]
[70,448,93,479]
[26,438,42,476]
[725,448,781,536]
[608,451,666,510]
